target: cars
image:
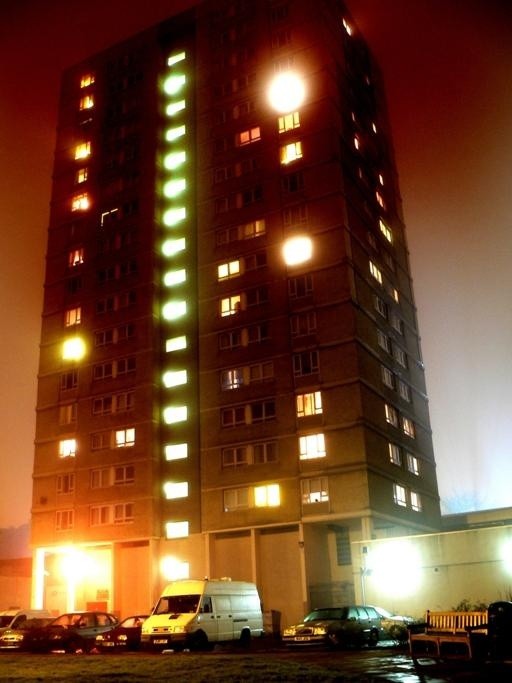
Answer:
[364,605,417,639]
[1,612,168,652]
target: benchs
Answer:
[406,608,490,659]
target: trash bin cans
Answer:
[488,601,512,660]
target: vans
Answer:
[0,608,54,634]
[140,577,265,647]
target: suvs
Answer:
[281,603,379,650]
